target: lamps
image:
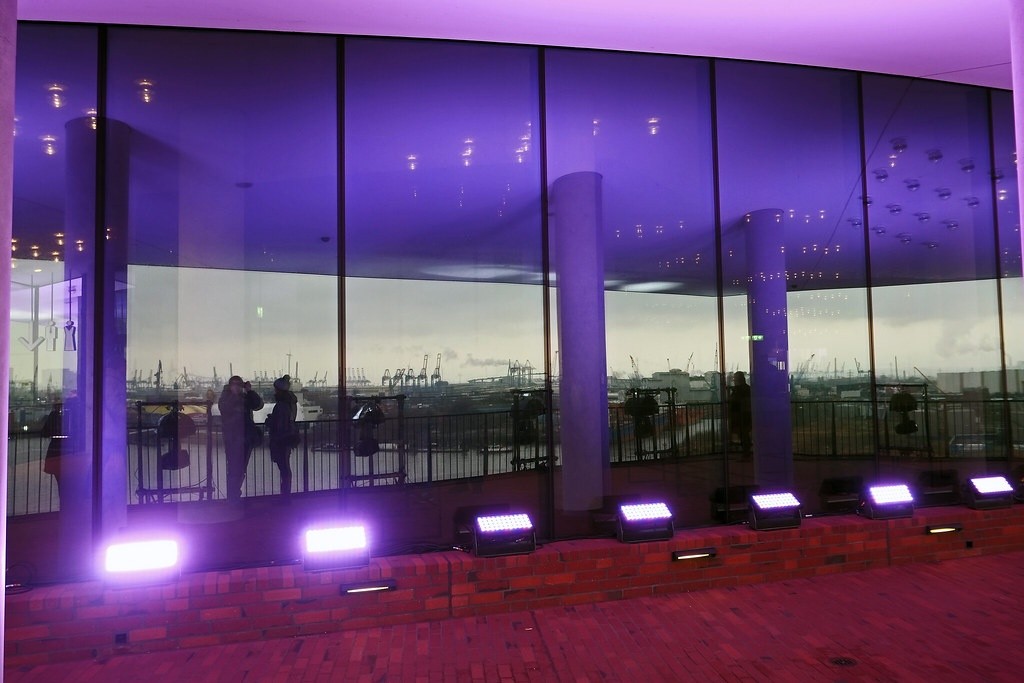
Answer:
[465,512,537,558]
[615,501,675,544]
[854,481,916,520]
[744,491,804,532]
[94,536,183,590]
[303,523,371,573]
[962,475,1018,510]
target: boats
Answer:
[481,443,511,452]
[378,442,410,452]
[417,442,470,453]
[311,442,337,453]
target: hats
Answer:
[274,374,290,391]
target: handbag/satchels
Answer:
[247,426,263,449]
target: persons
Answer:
[217,375,264,508]
[264,373,299,514]
[727,370,755,463]
[39,408,63,519]
